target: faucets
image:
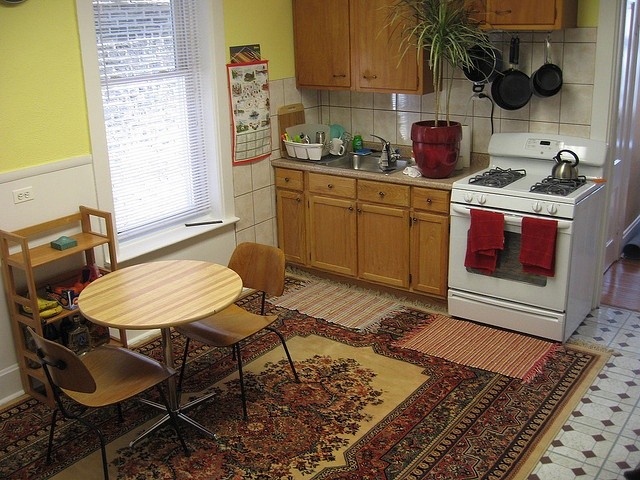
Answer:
[371,135,386,145]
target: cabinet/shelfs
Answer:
[274,167,307,272]
[448,0,578,31]
[411,186,450,302]
[292,0,443,95]
[307,173,411,304]
[0,204,129,409]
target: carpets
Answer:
[392,314,565,383]
[1,264,613,479]
[264,279,408,332]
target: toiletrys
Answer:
[353,135,363,152]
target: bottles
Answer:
[60,315,73,345]
[45,318,61,346]
[353,126,364,152]
[70,315,92,356]
[88,320,110,348]
[24,327,41,368]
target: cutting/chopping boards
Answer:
[278,102,306,158]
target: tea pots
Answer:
[551,149,580,180]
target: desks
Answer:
[78,260,243,450]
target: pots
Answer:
[491,34,533,112]
[462,34,503,92]
[533,38,563,97]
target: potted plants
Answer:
[380,0,507,179]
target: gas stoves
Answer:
[468,166,587,199]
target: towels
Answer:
[464,209,505,276]
[518,216,558,277]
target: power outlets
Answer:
[12,186,34,204]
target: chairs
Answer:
[178,242,302,421]
[25,325,191,479]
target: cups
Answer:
[316,131,325,144]
[330,124,344,141]
[328,138,345,156]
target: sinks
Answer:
[324,152,414,175]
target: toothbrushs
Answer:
[339,132,343,139]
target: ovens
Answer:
[448,190,607,344]
